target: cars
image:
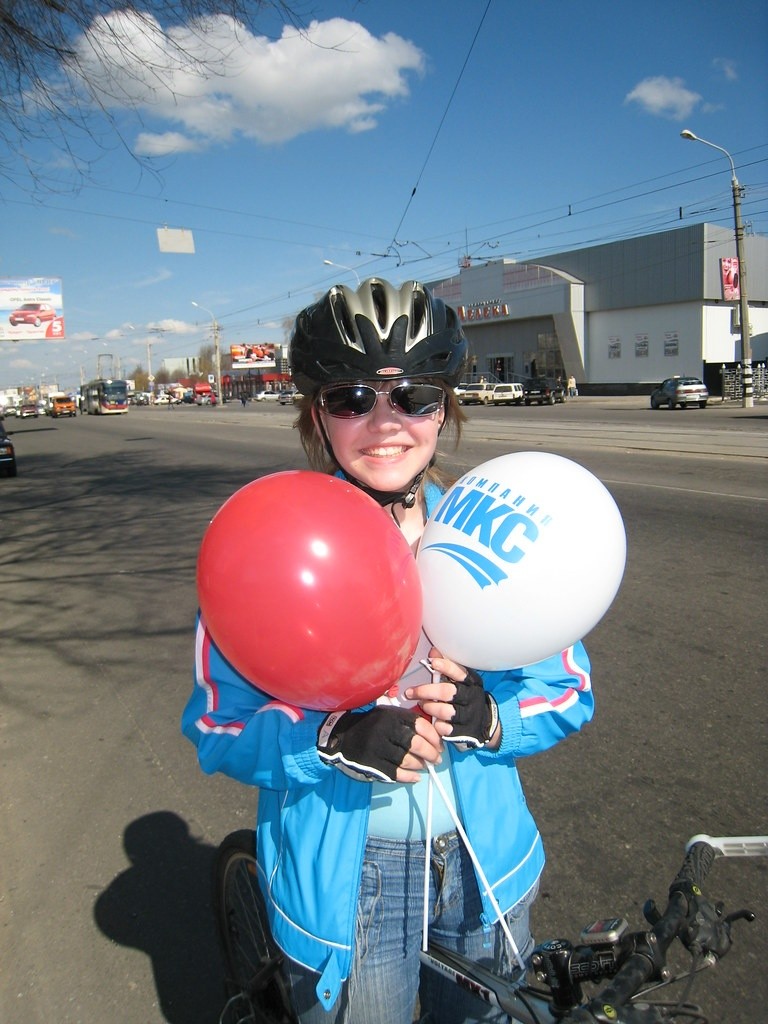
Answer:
[128,396,148,406]
[492,383,525,406]
[0,422,17,478]
[278,388,305,405]
[453,383,469,404]
[153,396,182,406]
[650,377,709,410]
[194,394,219,406]
[9,302,57,327]
[254,390,281,402]
[0,404,47,421]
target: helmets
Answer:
[287,278,469,395]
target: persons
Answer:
[179,278,595,1024]
[567,374,576,399]
[167,394,176,410]
[240,390,248,407]
[479,375,488,383]
[210,392,217,407]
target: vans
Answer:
[45,392,76,418]
[192,383,217,406]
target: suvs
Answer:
[523,377,566,406]
[460,383,497,405]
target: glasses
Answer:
[315,384,448,420]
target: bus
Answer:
[80,378,130,415]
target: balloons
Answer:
[414,449,627,672]
[195,467,424,713]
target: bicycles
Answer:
[209,828,768,1023]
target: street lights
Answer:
[679,128,754,408]
[128,325,153,393]
[191,302,223,406]
[322,259,360,287]
